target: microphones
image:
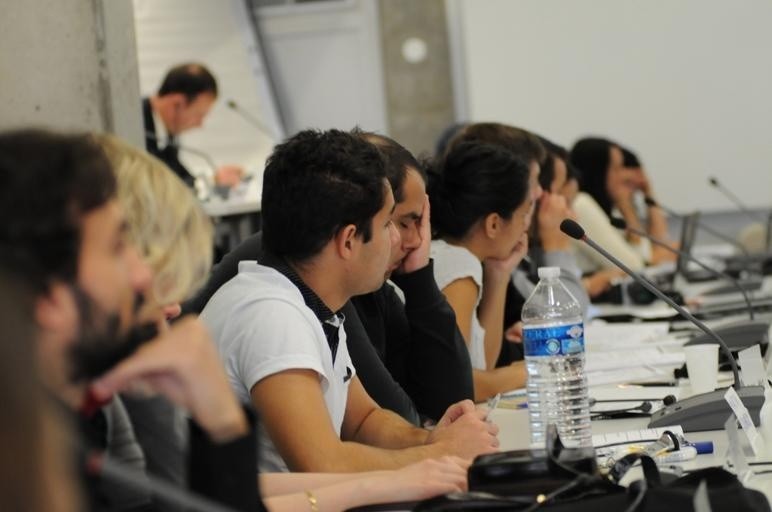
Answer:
[709,177,761,218]
[610,218,770,348]
[644,197,761,294]
[227,99,281,145]
[559,218,765,433]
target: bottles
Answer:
[520,265,594,451]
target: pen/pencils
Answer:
[483,392,501,422]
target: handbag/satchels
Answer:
[468,446,602,496]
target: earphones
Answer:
[174,101,179,109]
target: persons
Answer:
[140,63,244,187]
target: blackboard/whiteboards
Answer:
[132,2,386,213]
[441,1,771,218]
